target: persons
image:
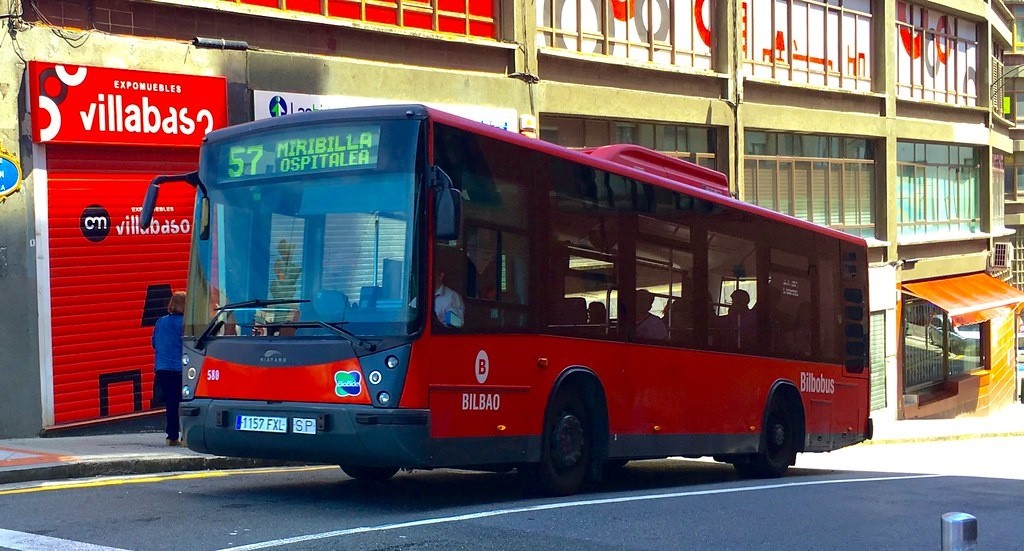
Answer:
[152,292,186,446]
[408,260,465,329]
[588,289,813,358]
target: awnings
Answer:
[901,273,1024,328]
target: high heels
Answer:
[166,437,180,447]
[181,438,186,447]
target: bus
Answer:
[138,105,873,495]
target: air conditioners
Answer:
[993,242,1011,268]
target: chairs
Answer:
[312,289,351,317]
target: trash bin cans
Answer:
[940,512,978,551]
